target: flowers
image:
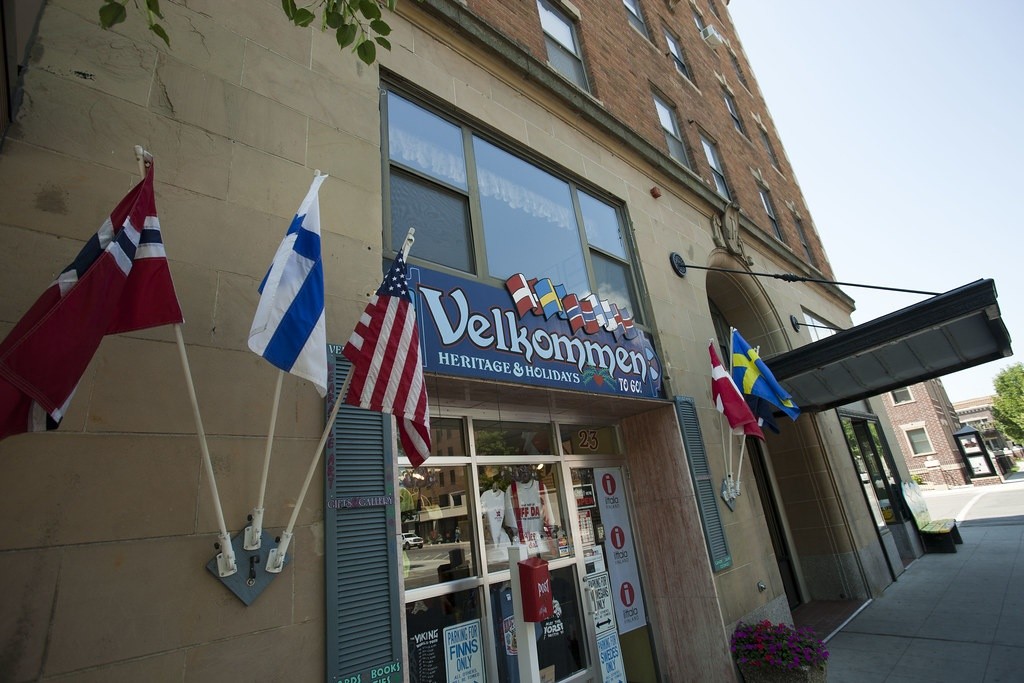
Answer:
[731,618,830,672]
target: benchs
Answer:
[900,480,964,553]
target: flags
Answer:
[341,245,432,469]
[708,329,799,443]
[0,156,185,441]
[247,174,328,396]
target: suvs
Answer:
[401,532,424,550]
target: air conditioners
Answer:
[701,24,723,50]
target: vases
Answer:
[739,661,828,683]
